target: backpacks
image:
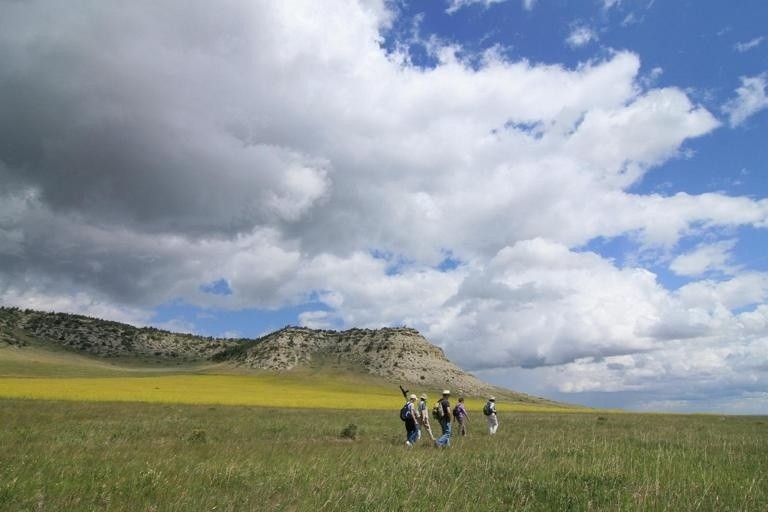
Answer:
[484,405,492,416]
[432,400,449,420]
[453,403,461,417]
[400,402,413,422]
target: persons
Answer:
[453,398,471,436]
[417,394,437,440]
[400,394,419,446]
[483,397,498,434]
[432,390,452,448]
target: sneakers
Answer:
[405,440,412,447]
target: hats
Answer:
[420,394,427,399]
[442,389,450,395]
[410,394,416,399]
[489,397,495,401]
